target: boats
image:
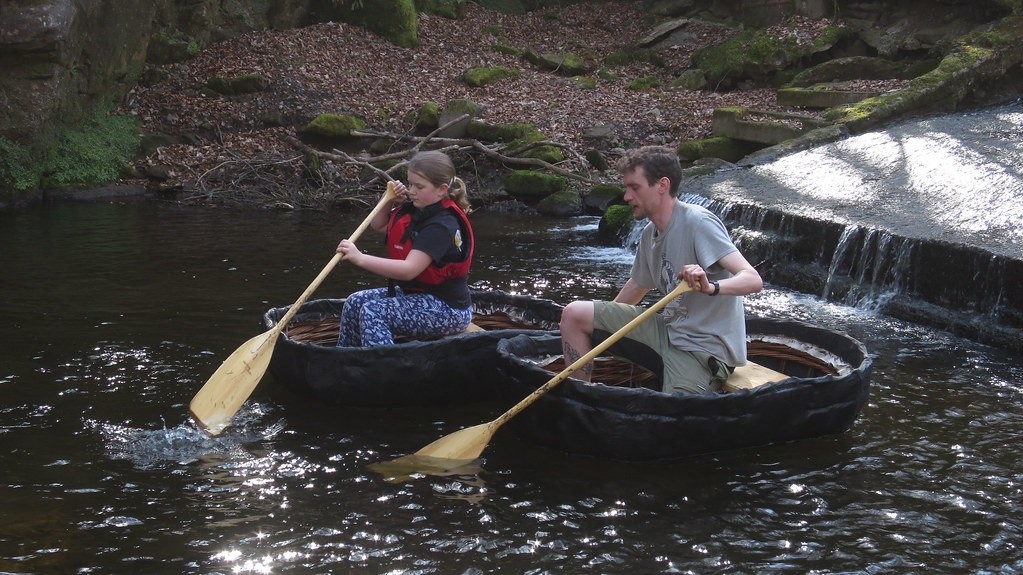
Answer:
[492,313,875,459]
[263,287,566,421]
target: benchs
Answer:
[727,361,790,391]
[442,322,487,341]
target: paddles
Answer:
[412,275,702,459]
[187,179,403,437]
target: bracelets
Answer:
[709,281,719,297]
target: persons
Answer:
[560,145,763,394]
[336,151,473,348]
